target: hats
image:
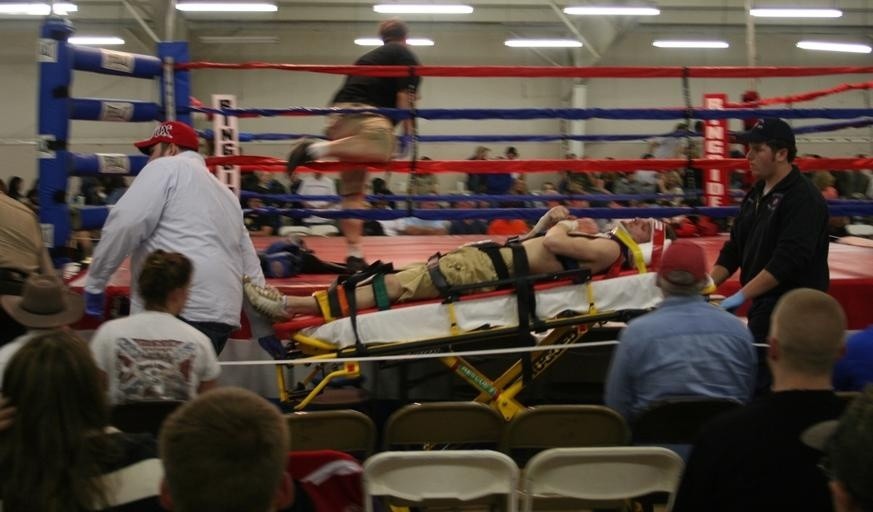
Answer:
[134,121,199,155]
[1,273,85,326]
[655,241,707,283]
[735,117,796,148]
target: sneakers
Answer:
[242,275,294,325]
[289,143,313,173]
[345,256,371,272]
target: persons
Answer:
[830,382,872,512]
[240,204,676,325]
[0,177,81,345]
[793,154,872,243]
[0,330,120,512]
[364,124,750,235]
[0,271,85,388]
[195,132,344,235]
[87,249,224,406]
[667,288,854,512]
[281,16,423,262]
[149,386,296,511]
[704,117,830,354]
[598,236,764,424]
[1,173,126,249]
[83,120,294,369]
